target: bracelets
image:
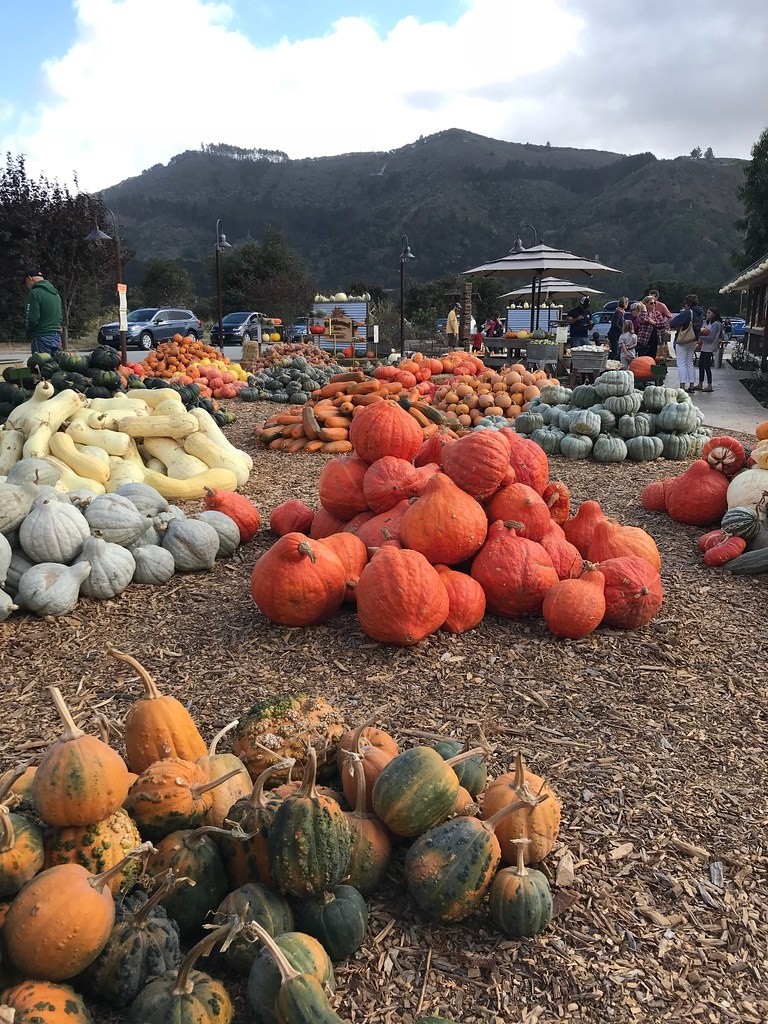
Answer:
[628,346,629,350]
[625,352,628,354]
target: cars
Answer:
[720,315,747,338]
[209,312,275,345]
[591,313,633,342]
[592,300,638,324]
[283,317,314,341]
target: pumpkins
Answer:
[473,356,711,463]
[0,640,560,1024]
[253,292,497,454]
[643,421,768,575]
[511,299,564,308]
[0,333,264,622]
[251,399,663,644]
[433,358,559,436]
[505,327,546,339]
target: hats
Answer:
[20,269,43,287]
[580,296,592,309]
[454,303,462,309]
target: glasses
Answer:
[629,309,636,312]
[647,302,656,305]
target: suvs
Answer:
[97,306,205,351]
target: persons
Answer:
[565,296,595,373]
[669,294,732,393]
[607,290,673,372]
[590,332,609,347]
[22,268,63,357]
[471,311,504,354]
[446,303,462,349]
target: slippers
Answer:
[693,386,702,390]
[702,388,714,392]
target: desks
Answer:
[482,336,563,358]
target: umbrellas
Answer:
[461,240,624,332]
[496,276,605,332]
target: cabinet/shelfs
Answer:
[256,324,285,357]
[295,315,378,359]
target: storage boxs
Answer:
[563,350,609,370]
[525,343,560,361]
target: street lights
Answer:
[400,234,415,357]
[508,224,539,333]
[83,207,127,367]
[215,218,232,357]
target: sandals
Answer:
[680,387,687,393]
[686,387,694,393]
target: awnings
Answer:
[719,258,768,294]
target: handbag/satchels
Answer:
[661,327,671,342]
[676,309,695,344]
